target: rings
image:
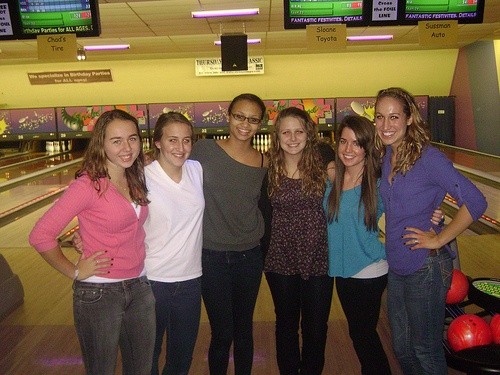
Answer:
[414,240,418,243]
[441,215,443,218]
[96,260,98,264]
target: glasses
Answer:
[228,109,261,124]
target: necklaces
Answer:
[117,184,127,191]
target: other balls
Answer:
[446,269,469,304]
[489,313,500,344]
[448,314,492,352]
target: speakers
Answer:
[220,32,248,71]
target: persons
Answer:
[327,88,488,375]
[324,114,446,375]
[188,93,271,375]
[73,110,205,375]
[263,108,334,375]
[27,109,156,375]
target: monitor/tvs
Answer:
[0,0,102,39]
[370,0,485,27]
[284,0,371,29]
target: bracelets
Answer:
[73,270,79,281]
